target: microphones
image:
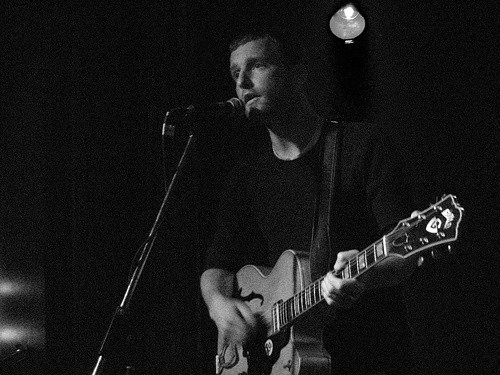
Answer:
[190,97,245,115]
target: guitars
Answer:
[213,193,465,375]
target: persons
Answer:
[199,28,425,375]
